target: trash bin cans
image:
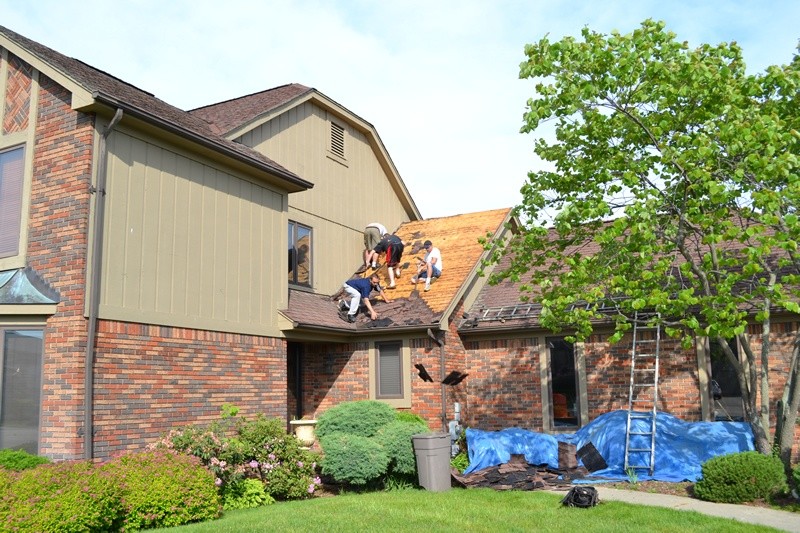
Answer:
[414,434,450,492]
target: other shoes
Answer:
[366,263,371,269]
[424,284,430,291]
[386,285,396,289]
[430,276,437,283]
[372,264,376,270]
[337,300,344,311]
[347,314,355,322]
[397,273,400,277]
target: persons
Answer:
[363,223,388,270]
[365,233,405,289]
[410,240,442,292]
[337,273,395,323]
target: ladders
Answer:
[475,297,629,320]
[624,312,660,475]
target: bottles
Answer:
[573,400,576,416]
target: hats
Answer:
[422,240,432,248]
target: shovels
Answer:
[410,265,419,298]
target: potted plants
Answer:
[289,417,318,451]
